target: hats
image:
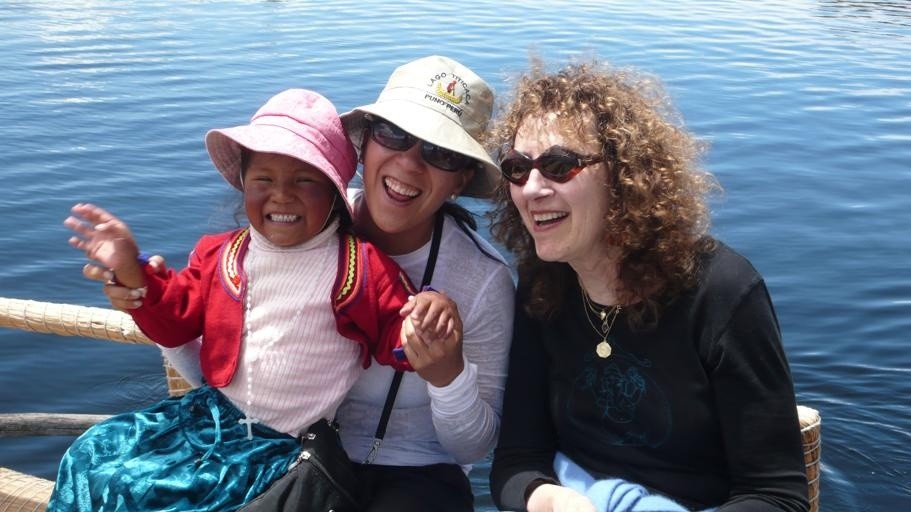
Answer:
[338,55,503,198]
[204,88,358,225]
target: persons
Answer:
[82,56,514,512]
[485,54,811,511]
[46,87,461,512]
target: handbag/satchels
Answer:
[234,418,363,512]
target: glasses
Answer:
[499,145,613,187]
[363,118,477,172]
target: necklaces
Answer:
[579,277,623,358]
[238,237,333,440]
